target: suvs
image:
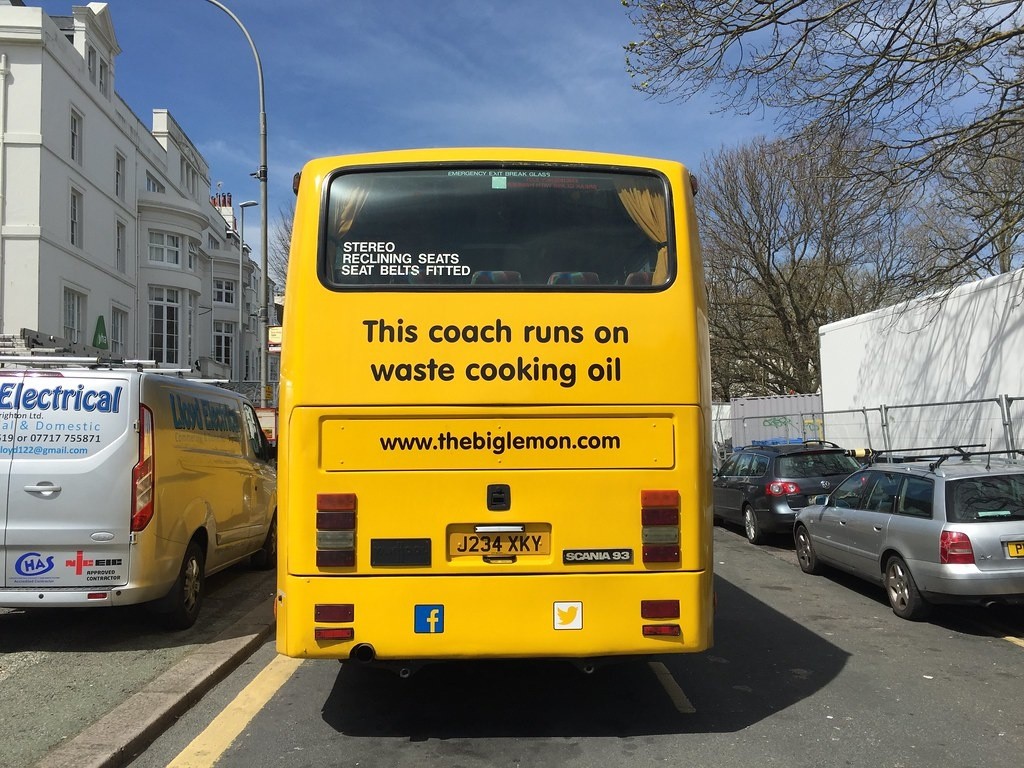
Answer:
[794,444,1023,623]
[713,438,865,546]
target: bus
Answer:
[274,146,714,670]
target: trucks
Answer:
[0,328,278,631]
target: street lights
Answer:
[239,199,260,392]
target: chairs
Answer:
[548,271,600,284]
[469,270,522,283]
[624,271,654,284]
[398,268,441,282]
[335,264,390,282]
[876,484,897,512]
[755,462,767,476]
